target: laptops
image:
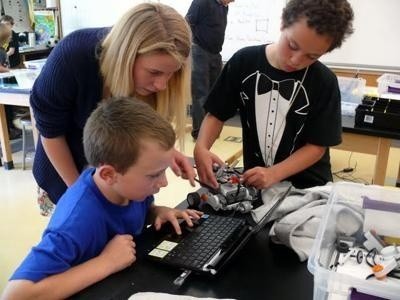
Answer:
[143,183,295,277]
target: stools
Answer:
[12,116,33,169]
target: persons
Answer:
[0,22,24,141]
[26,0,198,219]
[191,0,357,194]
[183,0,238,143]
[1,93,205,300]
[0,14,22,68]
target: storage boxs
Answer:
[307,180,400,300]
[336,73,399,130]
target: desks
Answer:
[65,125,400,298]
[11,48,51,68]
[0,88,38,169]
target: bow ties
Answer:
[257,74,294,101]
